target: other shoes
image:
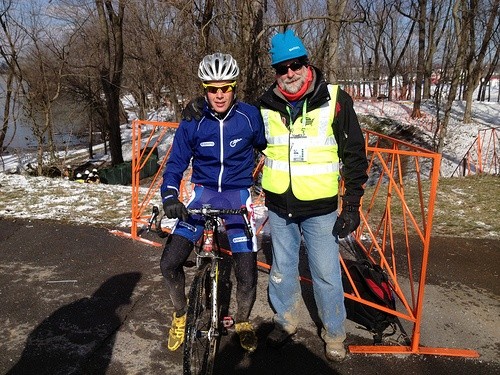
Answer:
[266,327,298,346]
[325,342,346,361]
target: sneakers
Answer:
[167,311,187,351]
[234,321,257,352]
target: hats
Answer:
[270,29,308,64]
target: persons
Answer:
[256,29,369,363]
[160,53,268,353]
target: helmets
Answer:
[197,53,239,81]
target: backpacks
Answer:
[339,260,397,329]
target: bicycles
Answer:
[146,205,254,375]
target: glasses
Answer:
[203,81,236,93]
[276,61,303,75]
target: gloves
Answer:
[332,206,360,238]
[161,190,188,219]
[181,97,211,122]
